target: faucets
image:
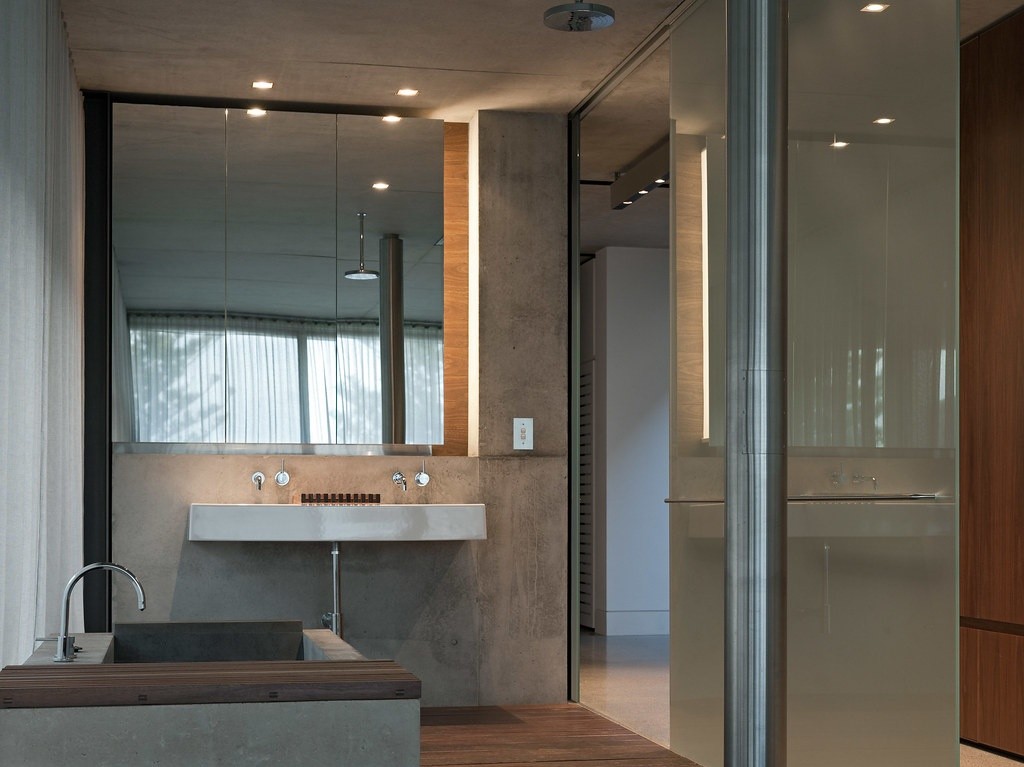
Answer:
[53,562,146,663]
[251,471,266,490]
[392,472,408,492]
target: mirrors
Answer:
[111,101,445,445]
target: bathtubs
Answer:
[0,621,422,767]
